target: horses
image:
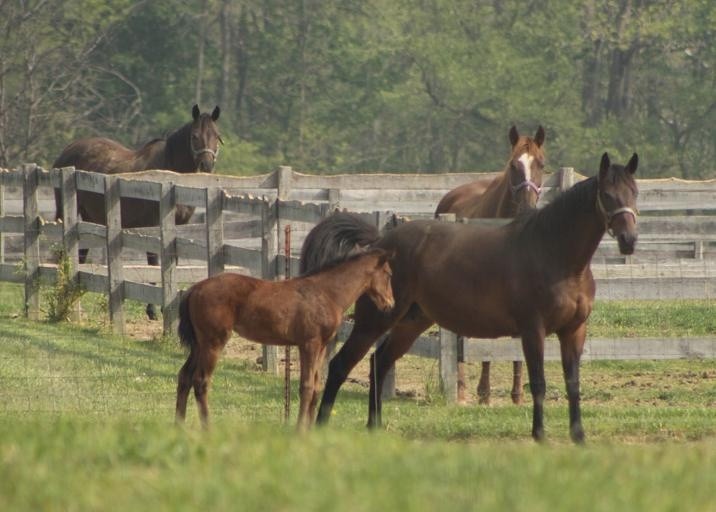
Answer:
[300,151,640,448]
[433,124,546,407]
[51,104,221,321]
[174,247,398,433]
